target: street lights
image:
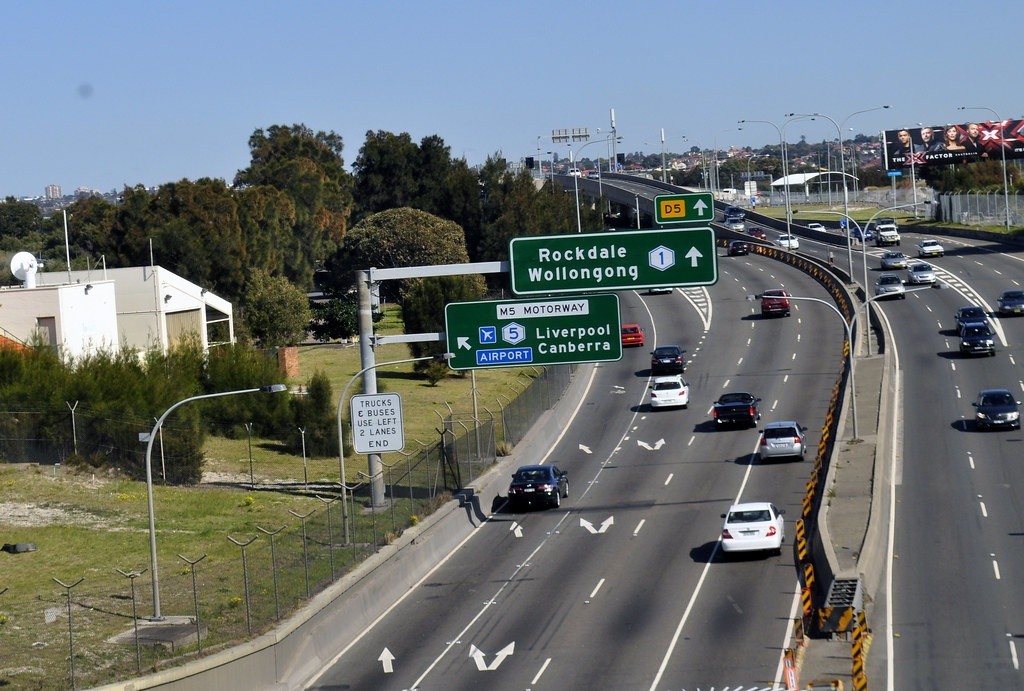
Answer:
[956,106,1012,231]
[785,199,941,357]
[762,283,950,443]
[145,381,288,621]
[337,351,457,543]
[537,103,894,281]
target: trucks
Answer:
[722,188,737,194]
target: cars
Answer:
[774,233,800,249]
[586,171,599,179]
[724,218,746,232]
[996,290,1024,316]
[971,389,1022,431]
[758,421,808,462]
[727,240,749,257]
[761,288,792,317]
[724,205,746,221]
[850,214,945,301]
[647,376,690,411]
[508,464,571,510]
[649,345,688,375]
[747,227,767,241]
[953,305,996,358]
[621,323,646,347]
[712,392,762,428]
[804,222,827,233]
[648,287,673,293]
[567,168,581,178]
[721,500,789,557]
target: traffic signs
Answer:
[444,296,622,372]
[510,225,719,294]
